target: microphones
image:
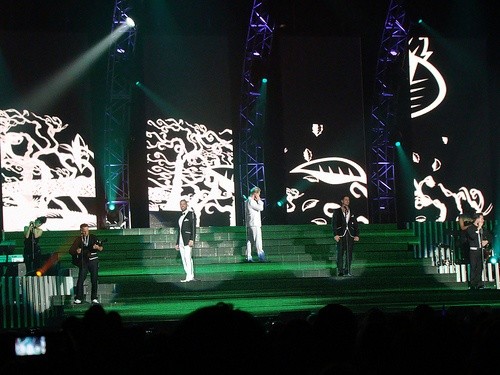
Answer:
[30,223,33,227]
[343,205,348,212]
[80,233,83,237]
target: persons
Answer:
[245,186,269,263]
[459,215,474,262]
[333,197,359,276]
[69,224,103,303]
[467,213,489,290]
[174,200,196,283]
[22,216,47,275]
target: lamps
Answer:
[24,269,42,277]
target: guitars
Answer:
[71,238,109,266]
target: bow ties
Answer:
[181,213,185,215]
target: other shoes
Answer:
[259,258,271,263]
[74,299,81,303]
[181,280,188,282]
[343,274,347,277]
[91,299,99,303]
[478,286,483,289]
[347,273,352,277]
[247,259,256,263]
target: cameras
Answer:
[8,327,49,362]
